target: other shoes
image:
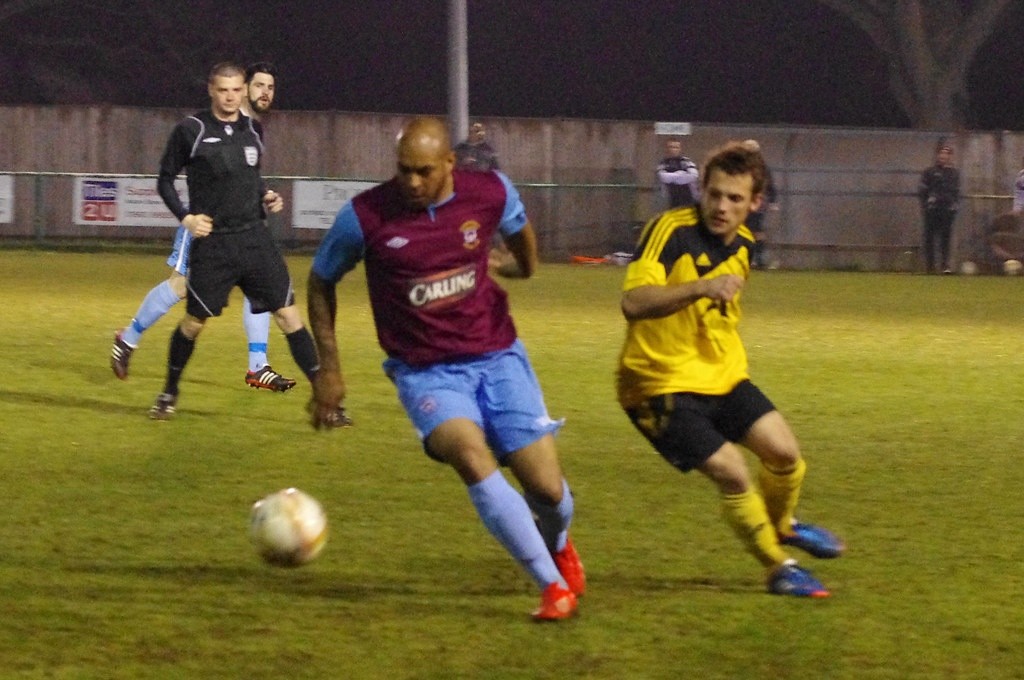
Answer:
[942,267,951,274]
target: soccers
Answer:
[251,487,330,567]
[960,262,979,275]
[1003,259,1023,275]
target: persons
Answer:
[741,138,775,269]
[110,59,296,394]
[149,59,351,428]
[615,141,844,598]
[920,147,961,273]
[656,137,699,205]
[450,123,505,252]
[306,114,585,624]
[1014,158,1024,212]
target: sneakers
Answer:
[779,519,843,559]
[534,519,587,598]
[304,395,355,429]
[767,564,832,599]
[148,389,179,420]
[110,328,139,379]
[245,364,297,393]
[532,583,581,623]
